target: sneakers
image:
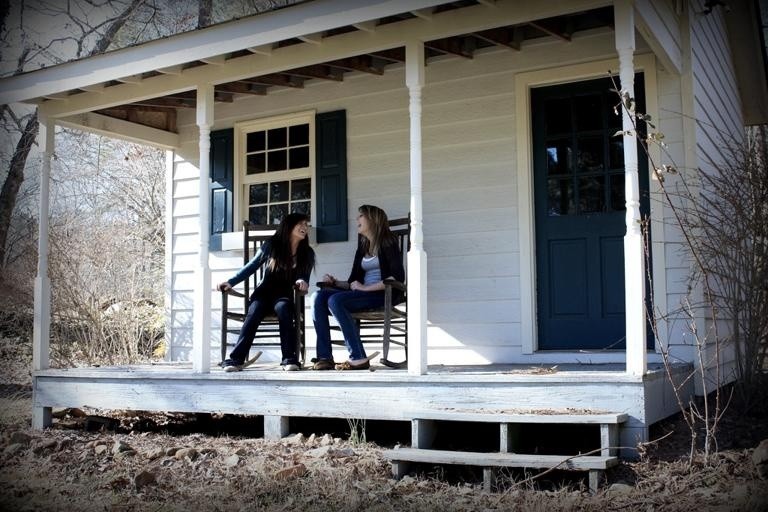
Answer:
[223,365,241,371]
[314,360,334,370]
[282,364,298,371]
[334,361,369,370]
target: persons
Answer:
[215,213,315,372]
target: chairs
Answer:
[219,219,304,369]
